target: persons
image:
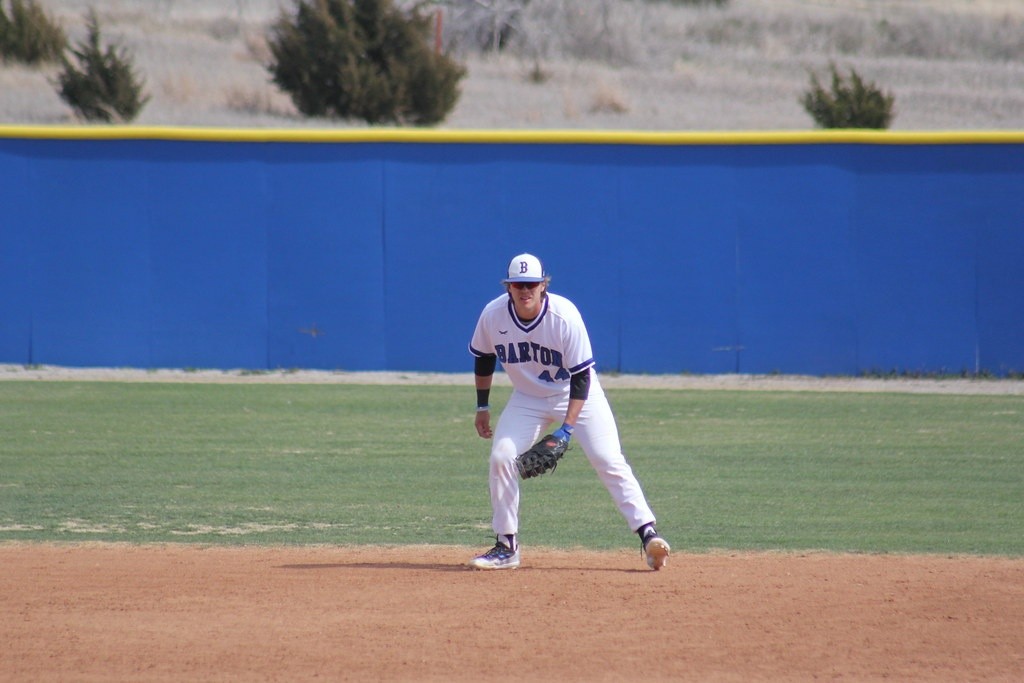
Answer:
[468,251,672,571]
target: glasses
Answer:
[508,282,540,290]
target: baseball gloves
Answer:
[515,433,568,480]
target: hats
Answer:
[506,251,546,282]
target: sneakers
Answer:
[468,542,520,571]
[640,530,671,569]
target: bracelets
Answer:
[476,406,490,411]
[477,389,489,406]
[562,423,575,435]
